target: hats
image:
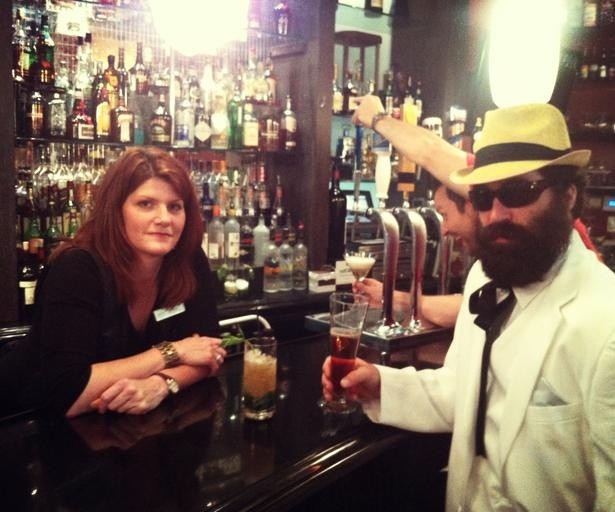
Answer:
[447,102,595,189]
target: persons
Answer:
[15,145,228,417]
[321,102,615,512]
[352,95,598,328]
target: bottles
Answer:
[331,59,485,270]
[573,1,615,80]
[364,0,384,21]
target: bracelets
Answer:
[372,112,389,130]
[153,340,181,367]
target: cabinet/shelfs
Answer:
[0,0,339,338]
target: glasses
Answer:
[465,176,564,210]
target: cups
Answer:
[314,291,371,418]
[238,336,278,423]
[558,107,615,137]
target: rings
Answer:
[216,355,222,360]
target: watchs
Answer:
[151,373,180,395]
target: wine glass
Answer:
[340,251,379,307]
